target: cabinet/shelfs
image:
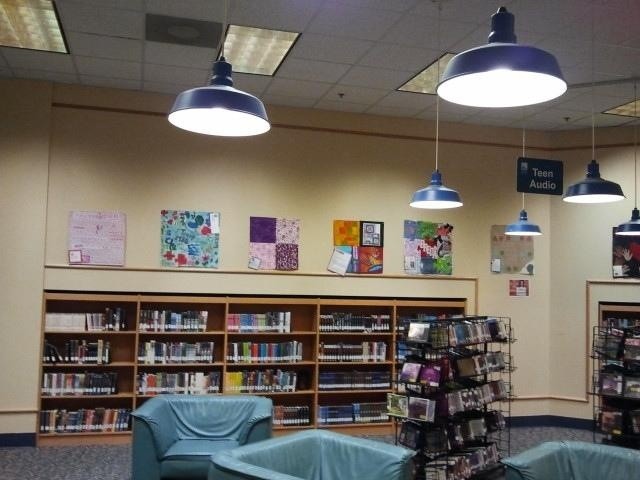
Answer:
[36,289,469,448]
[583,317,640,447]
[387,315,518,480]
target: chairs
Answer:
[208,428,417,480]
[500,440,640,480]
[128,393,275,479]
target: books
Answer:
[227,310,309,427]
[597,316,640,435]
[318,310,394,426]
[138,308,224,400]
[39,308,136,433]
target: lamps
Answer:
[435,6,568,108]
[503,102,545,236]
[408,0,465,211]
[613,80,640,236]
[561,1,627,205]
[165,0,273,139]
